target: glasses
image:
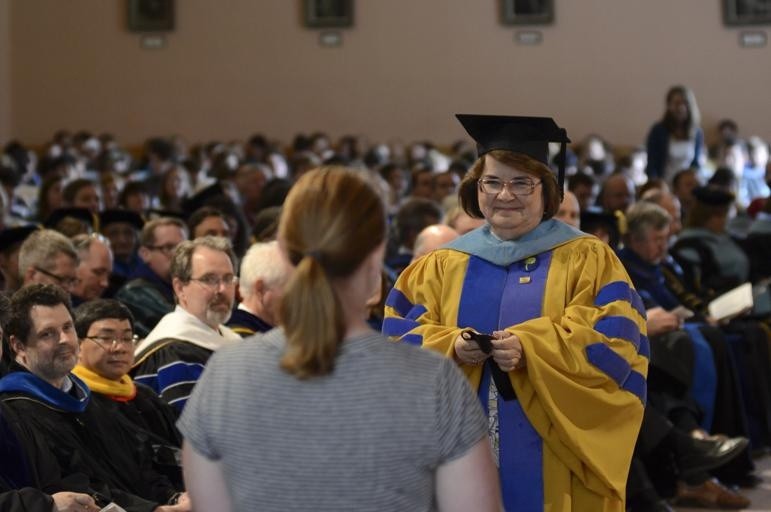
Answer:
[149,241,177,257]
[84,331,137,350]
[474,175,544,197]
[186,271,237,286]
[37,265,82,286]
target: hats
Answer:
[454,114,571,202]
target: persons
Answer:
[2,81,770,512]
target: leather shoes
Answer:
[683,430,748,484]
[676,478,750,509]
